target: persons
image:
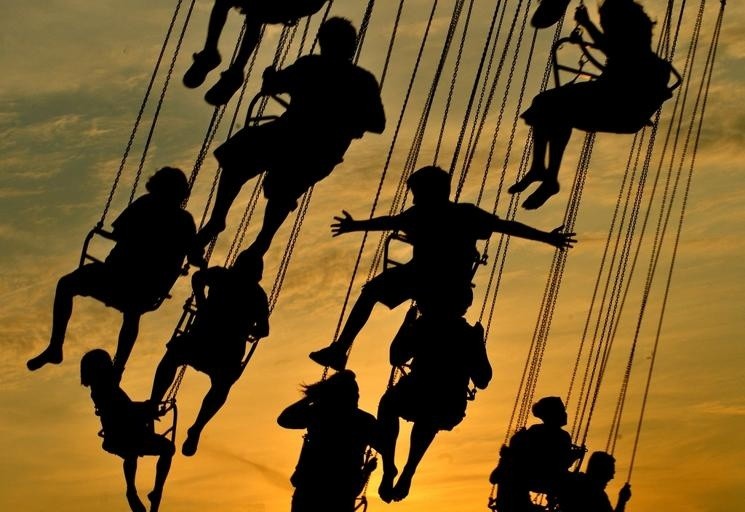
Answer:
[31,2,673,512]
